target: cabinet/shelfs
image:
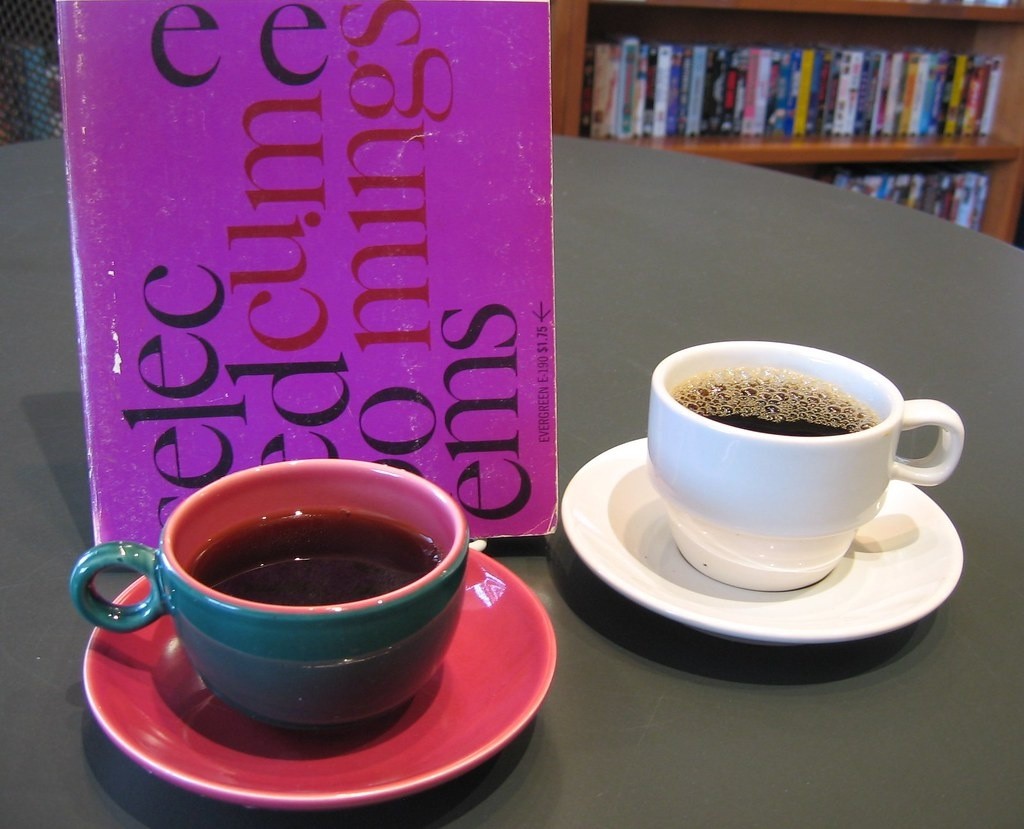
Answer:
[551,1,1024,251]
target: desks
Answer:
[0,135,1024,829]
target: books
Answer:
[579,33,1004,140]
[771,165,991,236]
[56,0,557,560]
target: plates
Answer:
[562,436,963,646]
[84,548,555,812]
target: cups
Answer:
[69,458,468,732]
[647,340,965,593]
